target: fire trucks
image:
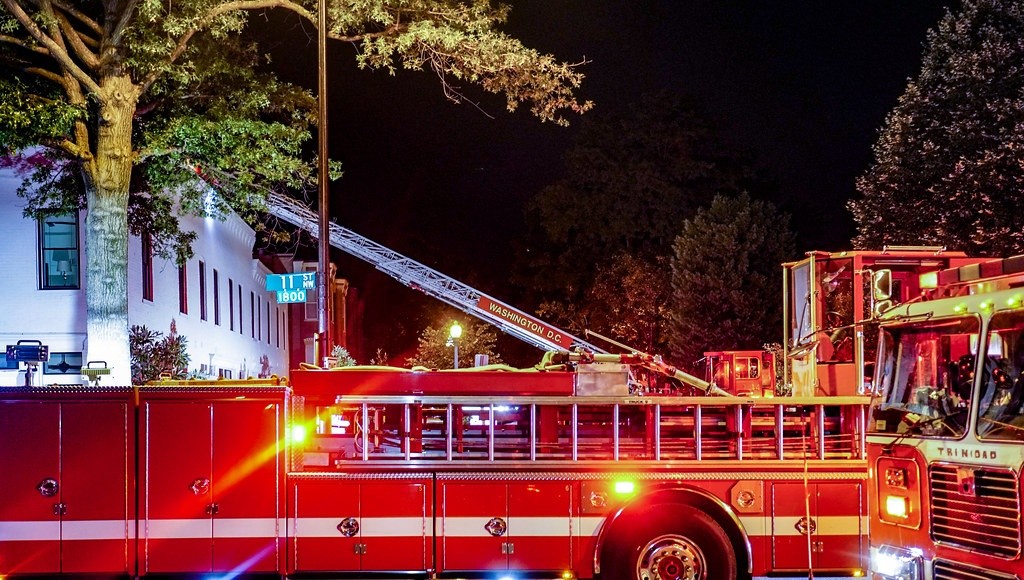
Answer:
[693,245,1002,397]
[1,160,876,580]
[855,254,1024,580]
